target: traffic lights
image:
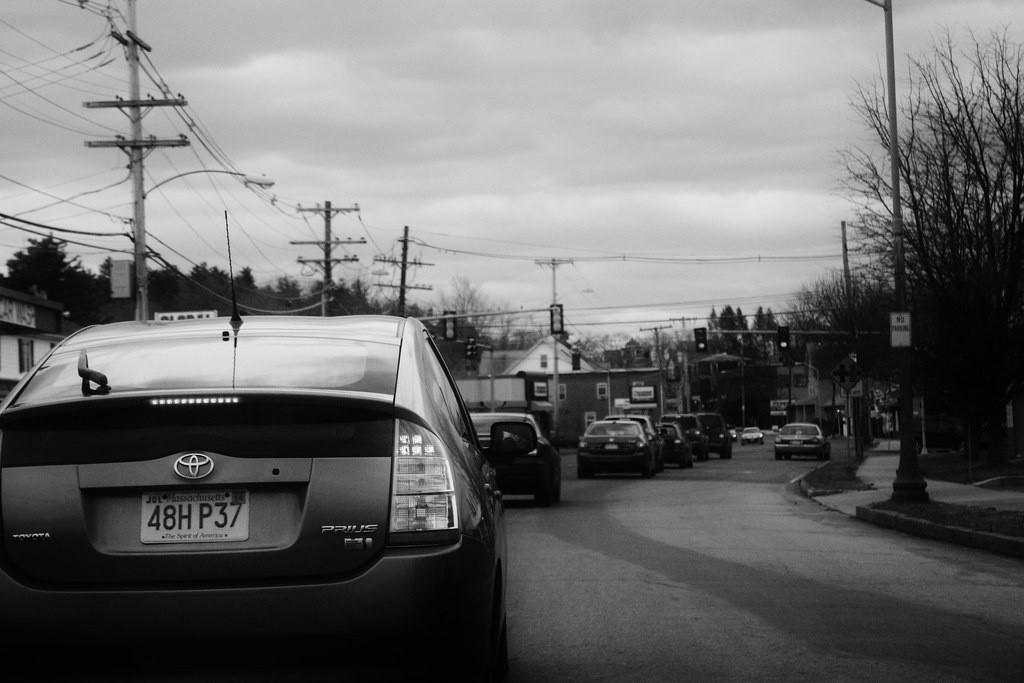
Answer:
[693,327,708,352]
[464,337,477,358]
[778,327,790,351]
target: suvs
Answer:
[660,414,707,461]
[604,413,664,474]
[697,412,734,459]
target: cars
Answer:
[470,411,563,502]
[577,421,653,477]
[655,424,694,468]
[773,422,830,459]
[0,314,540,683]
[741,427,764,445]
[727,424,736,439]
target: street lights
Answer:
[795,360,861,460]
[134,170,274,321]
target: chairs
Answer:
[595,428,606,435]
[623,428,634,435]
[660,428,667,434]
